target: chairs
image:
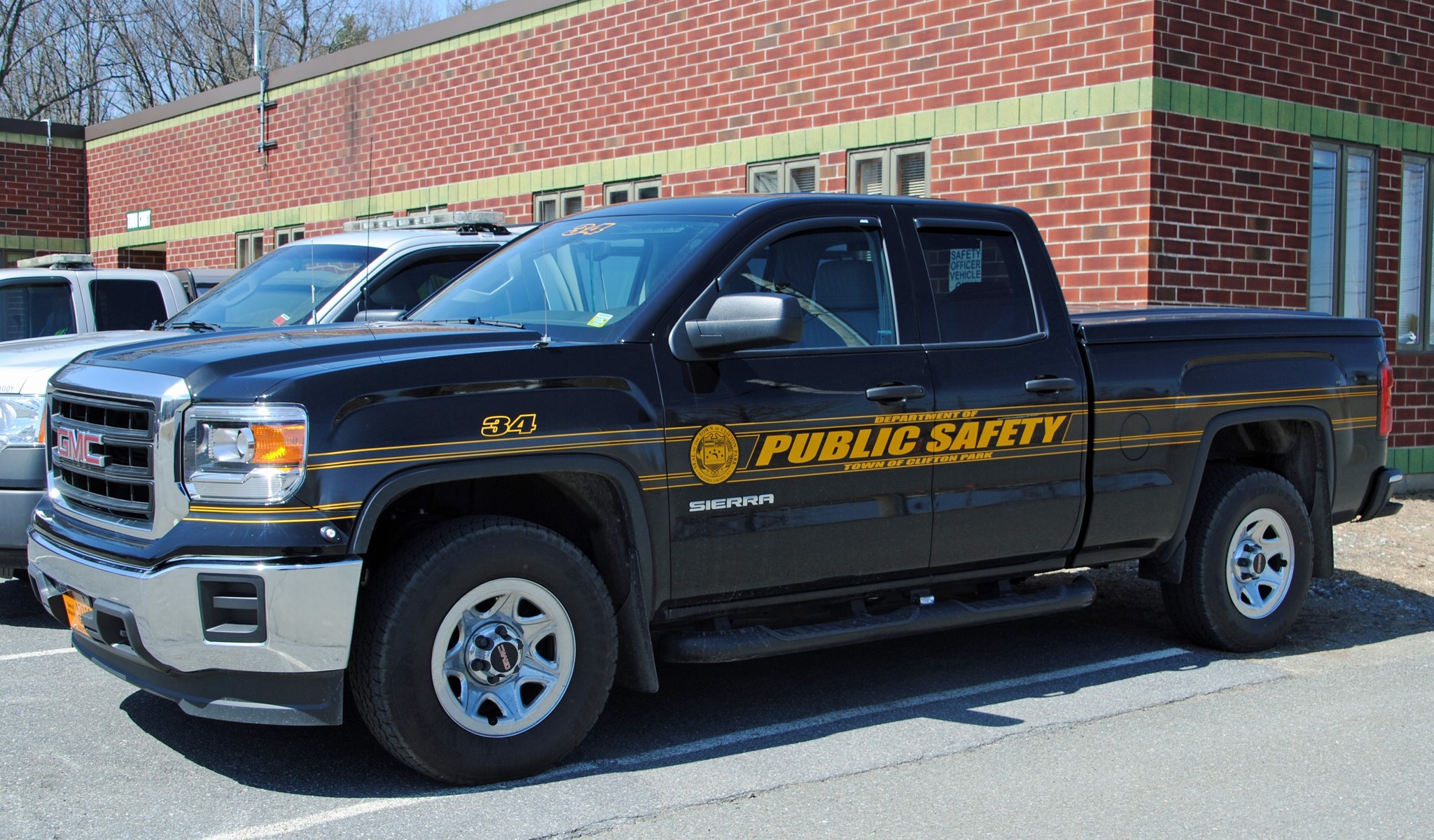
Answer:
[802,260,879,346]
[721,264,758,298]
[370,275,422,310]
[937,282,995,343]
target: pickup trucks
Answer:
[0,211,647,581]
[24,192,1405,786]
[0,253,339,342]
